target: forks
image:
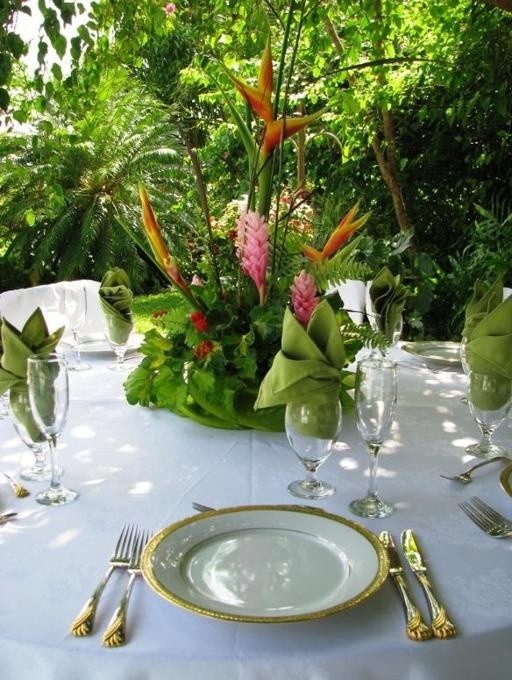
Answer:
[70,522,142,637]
[457,495,512,538]
[102,528,154,648]
[440,456,512,484]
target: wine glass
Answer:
[349,357,398,519]
[460,334,511,460]
[26,351,81,506]
[63,285,92,371]
[102,311,135,372]
[379,314,403,358]
[9,381,65,485]
[285,395,343,500]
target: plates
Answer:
[401,340,464,367]
[138,503,393,624]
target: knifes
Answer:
[379,530,434,642]
[401,527,457,641]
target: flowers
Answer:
[103,30,455,433]
